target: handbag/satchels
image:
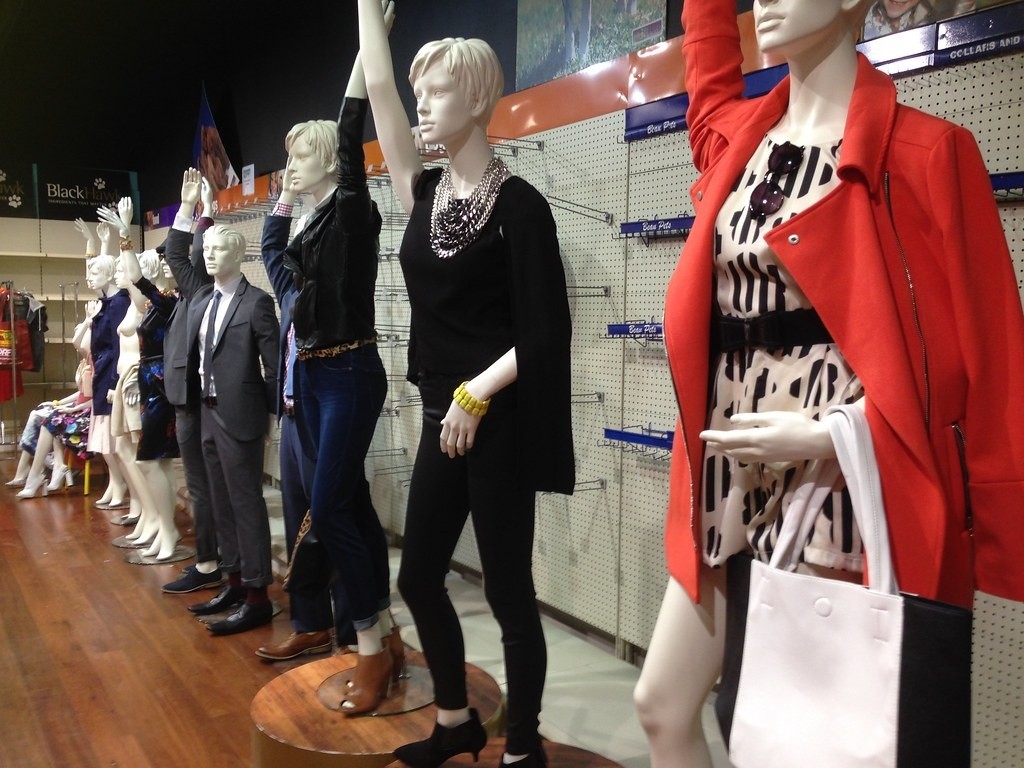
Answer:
[723,404,969,768]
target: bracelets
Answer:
[452,383,492,417]
[122,245,133,252]
[84,253,97,256]
[120,241,132,247]
[119,235,130,243]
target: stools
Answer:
[67,447,110,497]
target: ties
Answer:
[286,337,297,396]
[201,289,222,400]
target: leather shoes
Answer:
[205,602,273,635]
[187,585,246,614]
[255,627,332,661]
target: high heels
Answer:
[43,454,55,468]
[338,637,394,713]
[499,741,547,768]
[340,625,406,695]
[393,708,489,768]
[5,464,31,486]
[15,473,49,498]
[46,463,73,491]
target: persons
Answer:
[634,0,1024,768]
[257,160,352,662]
[357,0,575,768]
[164,167,286,634]
[280,1,400,717]
[1,176,215,563]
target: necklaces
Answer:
[427,157,506,259]
[143,288,171,309]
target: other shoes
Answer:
[121,513,140,525]
[162,569,222,594]
[181,553,223,573]
[120,516,128,520]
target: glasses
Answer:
[748,140,806,221]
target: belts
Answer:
[714,309,837,352]
